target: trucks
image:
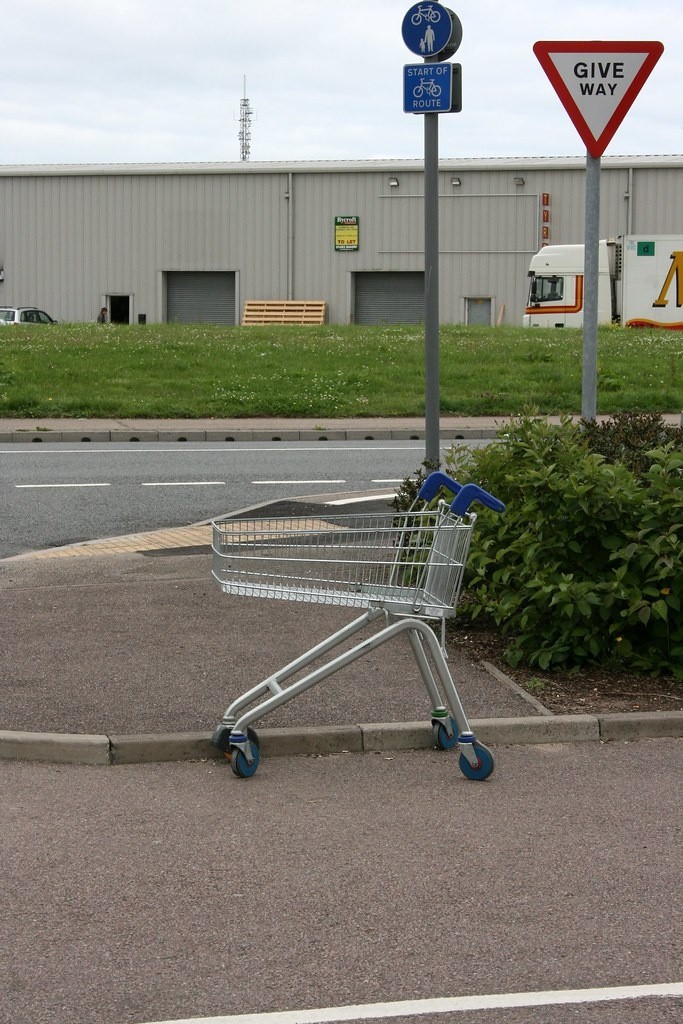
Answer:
[525,232,683,332]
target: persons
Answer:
[96,307,108,324]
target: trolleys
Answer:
[207,469,510,781]
